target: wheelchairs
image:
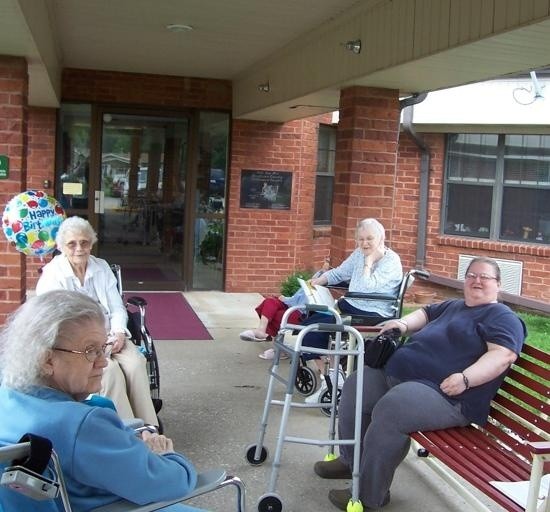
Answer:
[35,263,162,413]
[0,437,254,512]
[283,268,430,419]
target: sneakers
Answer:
[328,485,391,511]
[315,456,353,480]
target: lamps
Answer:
[347,39,361,53]
[257,84,268,91]
[167,24,193,34]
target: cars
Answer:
[112,166,164,204]
[197,167,227,196]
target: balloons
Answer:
[2,191,67,258]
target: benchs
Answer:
[353,270,550,512]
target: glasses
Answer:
[48,341,116,362]
[466,271,498,283]
[63,239,90,249]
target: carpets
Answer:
[122,291,214,339]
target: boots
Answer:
[316,367,345,402]
[305,375,328,403]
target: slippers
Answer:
[239,329,268,343]
[258,348,288,360]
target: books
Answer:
[297,277,337,315]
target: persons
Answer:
[239,255,333,361]
[34,215,159,434]
[313,254,530,512]
[1,289,211,512]
[293,216,405,405]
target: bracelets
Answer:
[399,318,410,333]
[364,262,372,270]
[461,371,471,390]
